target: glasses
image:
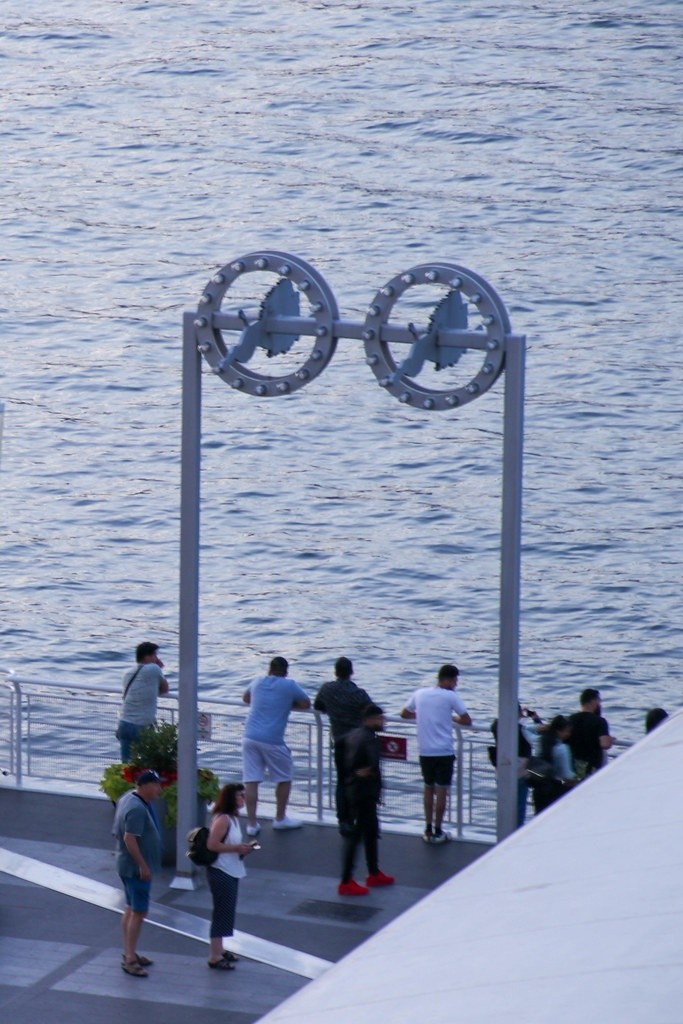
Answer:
[236,793,246,798]
[367,716,384,721]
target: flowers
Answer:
[97,720,219,823]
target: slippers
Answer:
[208,958,235,970]
[221,951,239,962]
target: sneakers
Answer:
[423,831,451,843]
[337,879,369,895]
[272,816,302,828]
[366,870,394,887]
[246,822,260,836]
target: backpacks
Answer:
[523,756,553,789]
[186,812,231,866]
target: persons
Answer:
[206,783,253,971]
[241,657,311,836]
[646,709,669,734]
[488,689,616,831]
[110,770,168,977]
[117,642,169,808]
[313,657,376,838]
[400,665,471,843]
[336,704,395,895]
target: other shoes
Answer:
[338,825,352,837]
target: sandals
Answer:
[121,960,149,977]
[121,953,153,967]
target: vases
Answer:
[113,795,208,868]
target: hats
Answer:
[137,770,167,786]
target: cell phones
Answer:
[527,710,535,717]
